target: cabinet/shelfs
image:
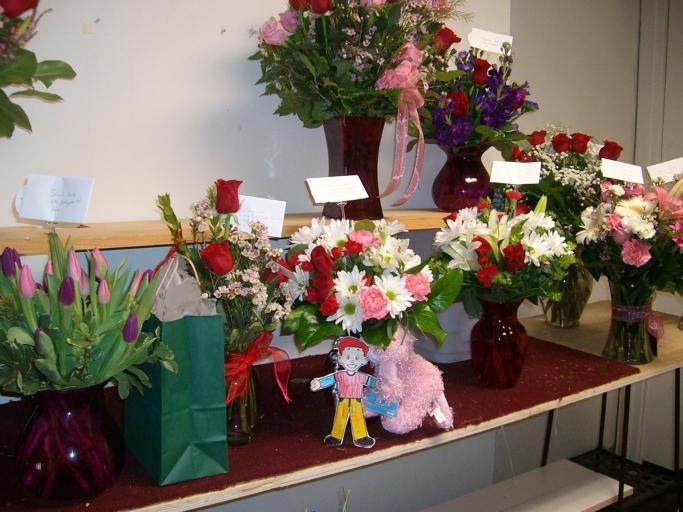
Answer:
[0,209,683,512]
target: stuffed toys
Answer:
[359,326,455,435]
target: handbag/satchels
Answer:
[122,253,229,487]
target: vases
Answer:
[601,281,656,366]
[326,334,371,407]
[538,264,593,327]
[470,299,529,390]
[16,390,125,505]
[323,115,386,220]
[431,142,492,211]
[221,354,258,445]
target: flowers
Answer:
[431,189,577,319]
[248,0,461,127]
[155,178,285,354]
[575,173,683,298]
[510,126,623,257]
[0,0,78,143]
[406,42,539,163]
[275,217,463,351]
[0,230,178,399]
